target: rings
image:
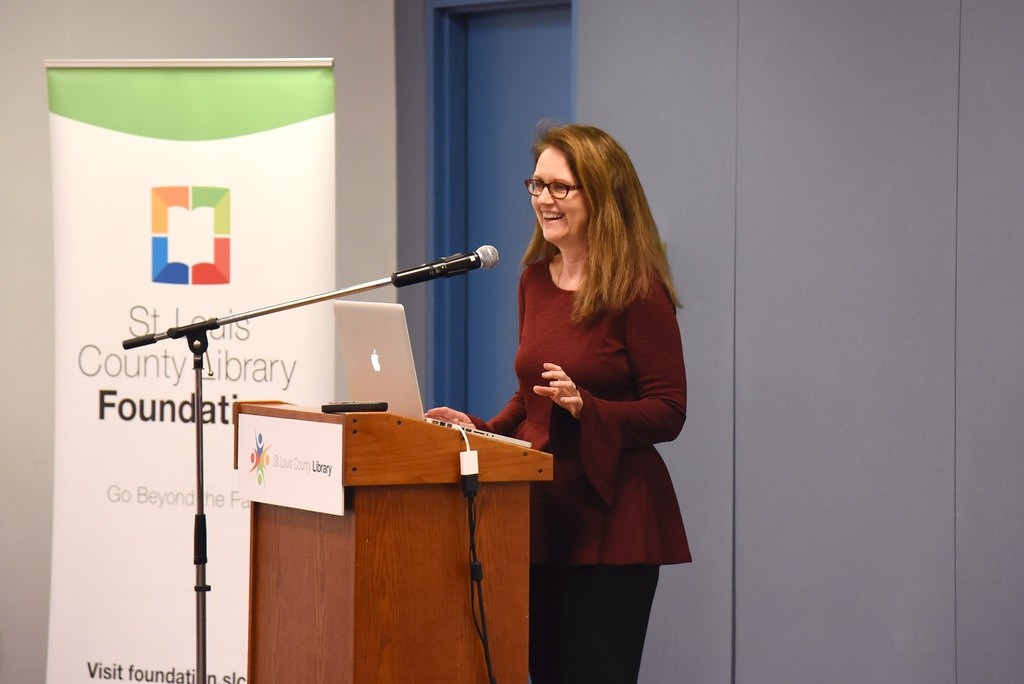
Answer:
[572,386,577,393]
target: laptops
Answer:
[333,301,532,449]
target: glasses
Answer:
[524,178,583,199]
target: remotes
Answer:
[320,401,388,413]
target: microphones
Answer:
[390,245,499,288]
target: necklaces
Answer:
[558,253,564,286]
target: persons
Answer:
[424,123,693,684]
